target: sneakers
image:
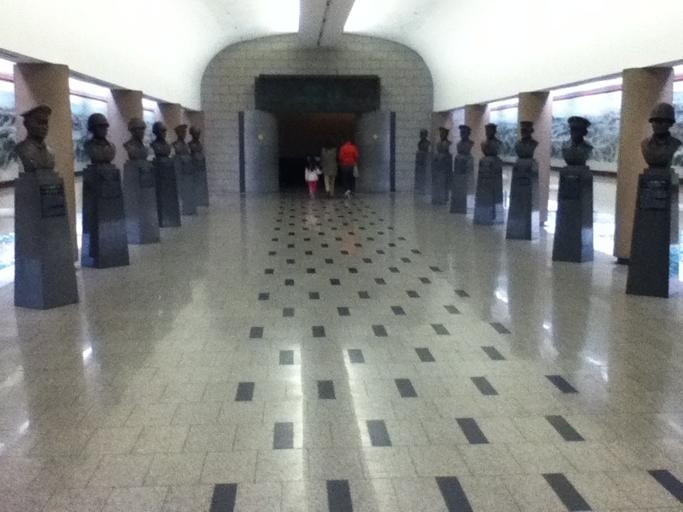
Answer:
[308,190,353,200]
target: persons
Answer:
[304,157,323,196]
[318,140,338,198]
[414,103,682,170]
[12,104,203,172]
[337,137,359,199]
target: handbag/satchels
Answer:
[353,165,359,179]
